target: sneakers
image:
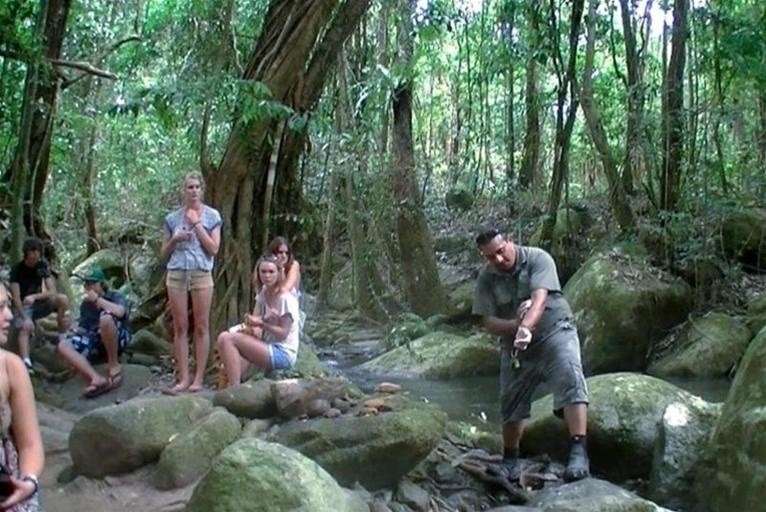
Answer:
[191,381,203,391]
[487,459,521,480]
[23,357,33,372]
[174,380,189,392]
[564,445,590,482]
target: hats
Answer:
[82,269,105,281]
[23,238,45,251]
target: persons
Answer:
[8,238,73,376]
[161,170,222,392]
[0,281,44,511]
[55,266,131,400]
[255,235,306,338]
[471,226,591,482]
[216,256,302,391]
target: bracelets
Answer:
[190,221,202,228]
[21,473,40,496]
[94,294,102,305]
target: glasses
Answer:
[277,251,289,256]
[476,229,500,244]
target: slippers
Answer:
[83,378,108,397]
[108,362,124,389]
